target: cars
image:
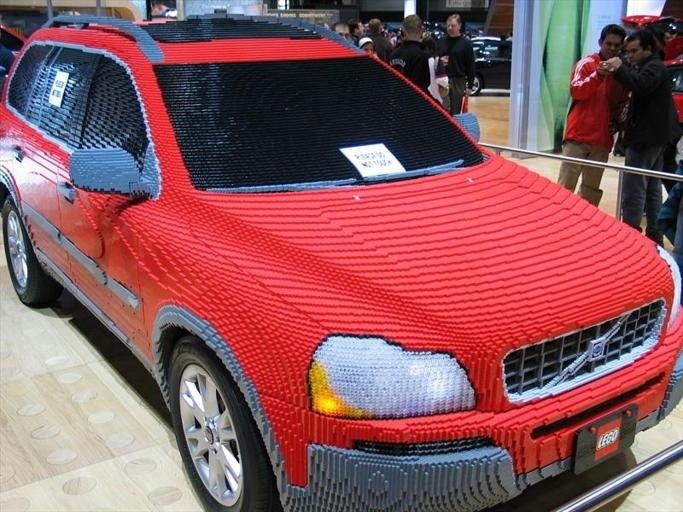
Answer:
[1,13,681,512]
[465,35,511,97]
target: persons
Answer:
[318,12,512,114]
[556,24,682,274]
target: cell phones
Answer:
[602,61,614,72]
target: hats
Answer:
[358,37,373,49]
